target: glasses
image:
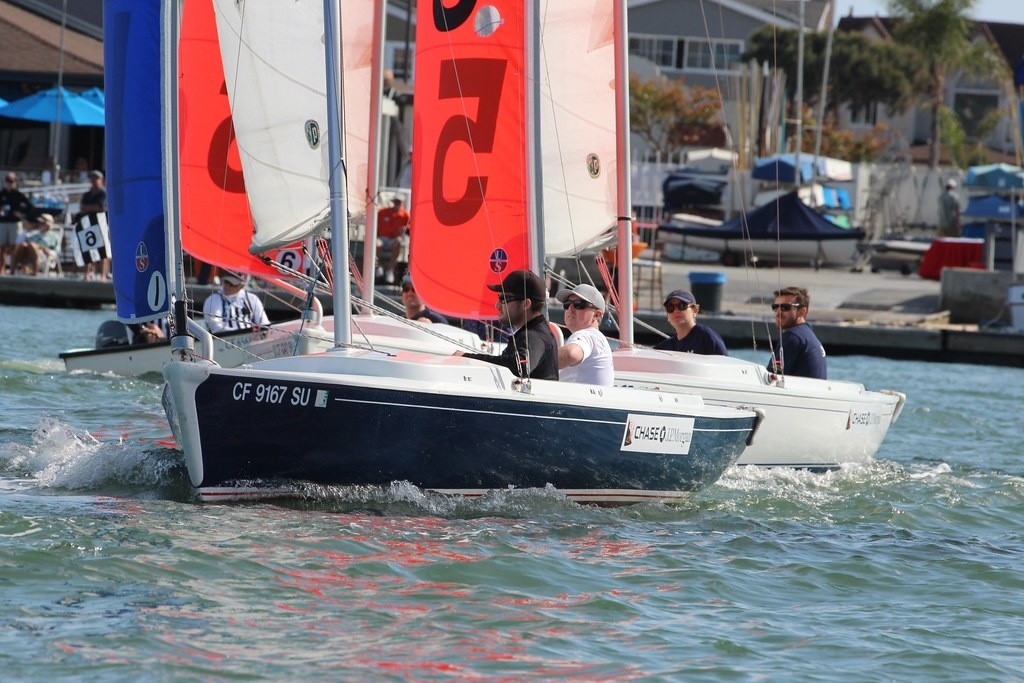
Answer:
[563,300,597,310]
[771,303,800,311]
[665,302,692,313]
[497,294,523,303]
[402,286,415,291]
[224,280,234,286]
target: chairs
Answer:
[40,225,65,276]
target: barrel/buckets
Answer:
[688,270,724,313]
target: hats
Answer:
[400,274,411,285]
[666,289,696,305]
[487,269,546,302]
[555,283,605,318]
[222,270,244,286]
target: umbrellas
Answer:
[0,84,105,186]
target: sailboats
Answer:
[57,0,908,508]
[659,0,866,269]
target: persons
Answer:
[202,269,271,333]
[9,214,60,276]
[0,171,27,275]
[937,179,961,237]
[401,270,449,324]
[126,316,170,344]
[652,289,729,356]
[555,283,615,386]
[377,193,410,285]
[79,169,107,281]
[766,287,828,380]
[452,270,560,381]
